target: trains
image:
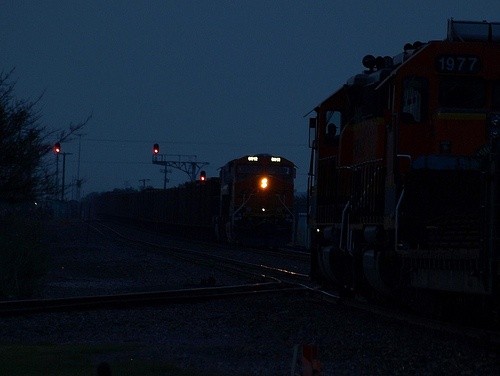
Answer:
[87,153,296,244]
[304,17,500,311]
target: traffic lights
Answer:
[54,143,61,153]
[153,144,159,155]
[200,170,206,182]
